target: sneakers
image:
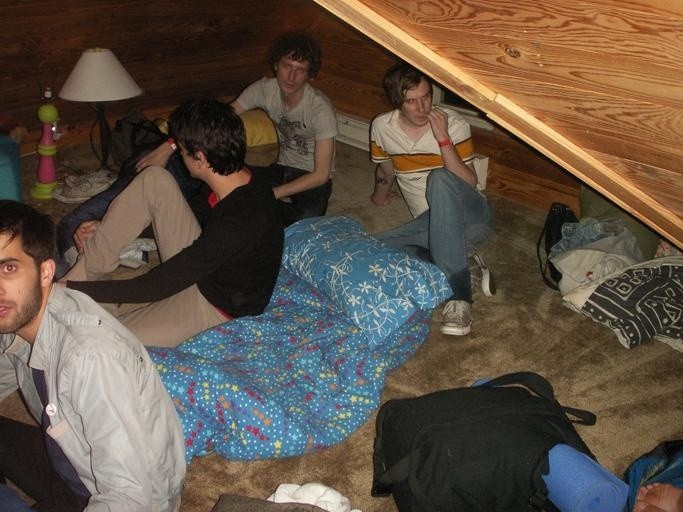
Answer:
[438,299,474,337]
[466,252,497,298]
[52,169,118,204]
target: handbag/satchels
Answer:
[372,369,598,512]
[109,104,169,169]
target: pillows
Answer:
[281,215,454,353]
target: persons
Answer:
[55,95,285,350]
[0,200,188,512]
[458,367,682,511]
[363,60,499,338]
[133,28,335,227]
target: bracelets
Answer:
[437,136,451,146]
[166,138,178,152]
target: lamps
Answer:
[58,48,143,163]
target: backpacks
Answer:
[536,202,580,292]
